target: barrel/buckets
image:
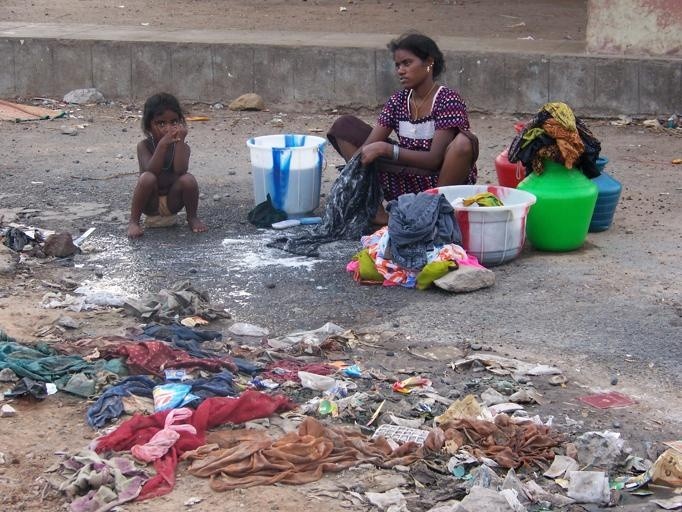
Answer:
[591,156,622,234]
[495,123,531,188]
[245,133,327,216]
[516,158,599,253]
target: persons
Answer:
[331,32,479,234]
[126,92,208,236]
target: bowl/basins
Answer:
[424,185,538,264]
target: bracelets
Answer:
[392,145,400,161]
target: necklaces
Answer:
[151,139,175,171]
[408,78,437,122]
